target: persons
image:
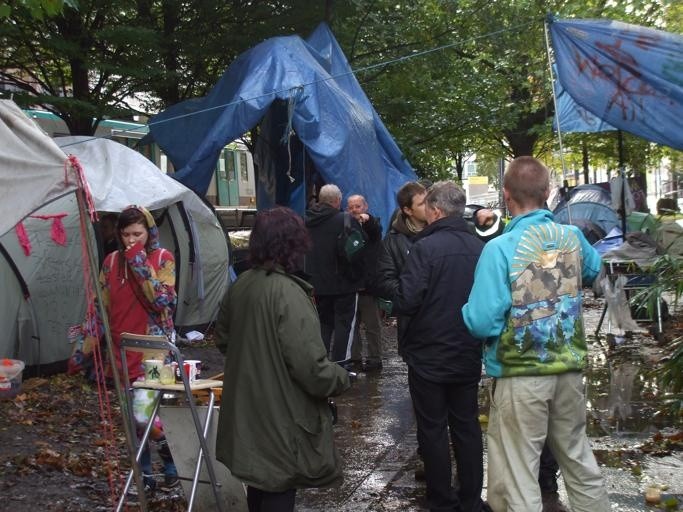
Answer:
[68,202,182,498]
[472,205,572,512]
[460,155,614,510]
[387,179,487,511]
[300,183,373,382]
[376,180,433,481]
[342,193,383,373]
[210,204,354,510]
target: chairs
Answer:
[116,333,224,511]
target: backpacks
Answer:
[335,211,375,285]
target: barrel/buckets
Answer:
[0,359,25,401]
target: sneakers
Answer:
[361,362,383,370]
[539,471,558,491]
[127,475,156,497]
[165,462,179,488]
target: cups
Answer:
[145,360,203,385]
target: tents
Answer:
[0,133,231,389]
[549,177,683,292]
